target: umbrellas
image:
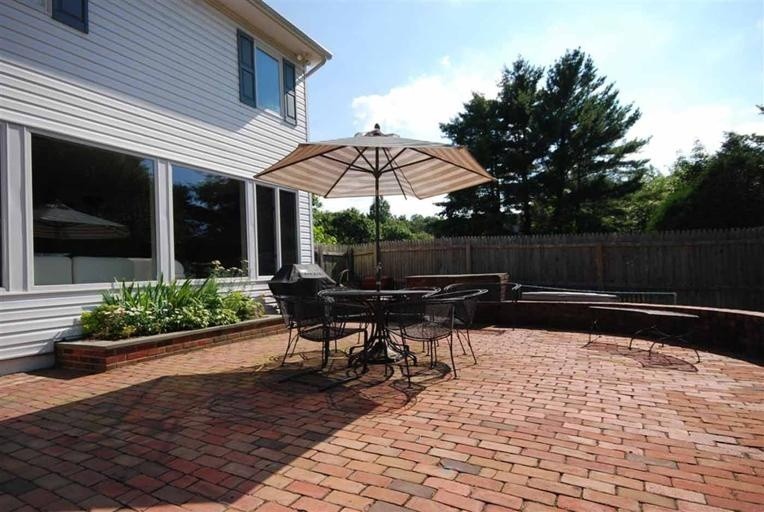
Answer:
[34,200,129,255]
[258,122,497,288]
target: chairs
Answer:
[272,282,488,389]
